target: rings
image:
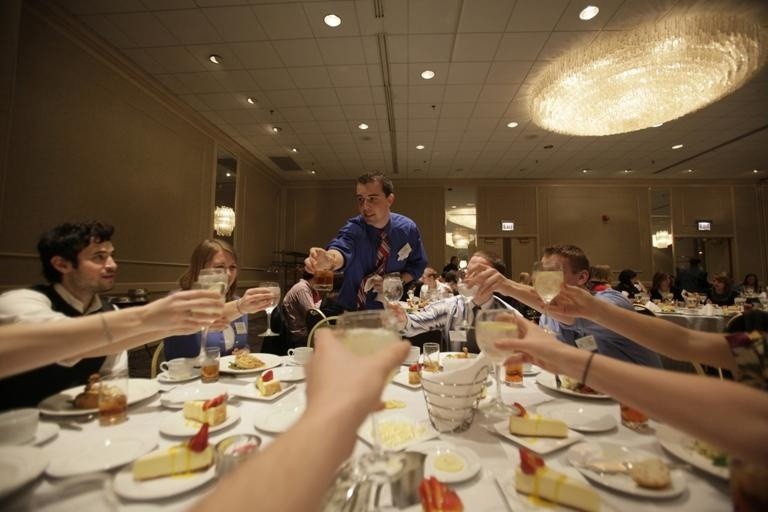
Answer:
[255,300,263,308]
[186,305,196,316]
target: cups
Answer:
[0,407,36,444]
[286,345,316,367]
[161,357,195,379]
[312,253,336,292]
[457,268,480,329]
[407,290,414,299]
[383,272,403,304]
[215,435,263,476]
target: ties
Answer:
[465,305,486,354]
[357,230,391,309]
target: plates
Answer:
[389,366,424,392]
[495,466,616,512]
[159,405,237,435]
[273,367,306,382]
[39,378,158,422]
[236,379,296,402]
[255,407,301,434]
[210,351,279,373]
[113,443,216,503]
[492,418,587,459]
[412,440,485,483]
[661,431,731,480]
[576,446,686,505]
[0,445,48,497]
[534,399,619,437]
[21,419,61,446]
[534,369,615,402]
[41,422,159,478]
[157,369,202,381]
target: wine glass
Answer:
[634,292,768,315]
[190,269,228,368]
[533,261,565,336]
[340,310,407,479]
[474,309,521,417]
[258,282,281,337]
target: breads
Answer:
[623,459,671,488]
[74,391,102,409]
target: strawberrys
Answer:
[418,477,463,511]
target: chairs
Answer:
[151,340,170,378]
[307,315,341,349]
[305,308,328,326]
[634,304,655,316]
[727,309,767,333]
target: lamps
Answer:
[652,230,672,249]
[214,206,235,237]
[452,227,470,246]
[529,12,768,138]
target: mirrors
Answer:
[648,186,677,276]
[443,186,478,267]
[209,142,240,245]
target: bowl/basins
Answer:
[108,287,151,305]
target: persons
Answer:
[280,253,460,337]
[1,290,226,380]
[463,246,665,369]
[162,236,277,368]
[0,214,132,412]
[491,311,768,464]
[186,325,411,511]
[531,280,767,512]
[304,170,427,311]
[377,250,526,352]
[591,259,767,302]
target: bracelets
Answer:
[581,351,594,387]
[235,296,247,318]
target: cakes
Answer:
[408,363,422,384]
[182,395,229,426]
[132,422,213,483]
[513,445,600,510]
[256,372,281,396]
[509,403,567,438]
